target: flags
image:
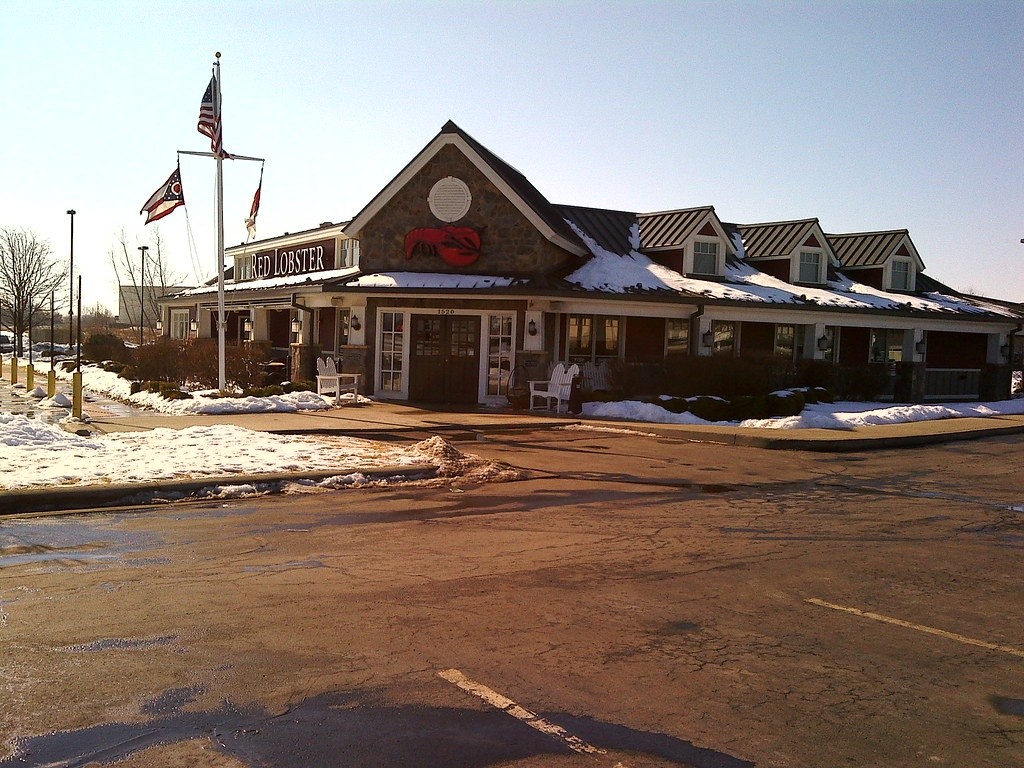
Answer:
[197,68,230,159]
[244,177,264,241]
[140,167,186,227]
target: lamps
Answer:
[292,317,302,332]
[1001,343,1010,356]
[916,339,925,353]
[703,330,714,347]
[818,335,828,351]
[529,319,537,335]
[156,319,163,329]
[191,318,199,331]
[351,314,361,330]
[244,318,253,332]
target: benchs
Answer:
[583,360,615,393]
[316,356,361,404]
[528,363,579,414]
